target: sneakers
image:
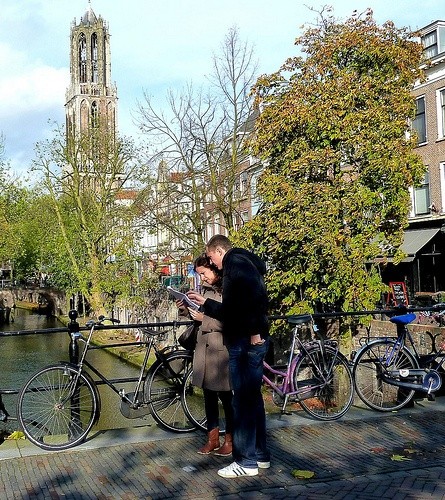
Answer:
[217,462,258,478]
[257,461,270,468]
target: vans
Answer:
[163,276,190,293]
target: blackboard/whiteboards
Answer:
[387,282,408,306]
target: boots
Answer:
[196,428,220,454]
[214,432,233,457]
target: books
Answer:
[167,286,200,312]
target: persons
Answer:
[175,253,233,457]
[185,234,271,479]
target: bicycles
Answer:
[178,315,355,435]
[347,303,445,412]
[16,315,209,452]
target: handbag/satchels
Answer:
[176,319,198,351]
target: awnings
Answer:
[362,228,440,263]
